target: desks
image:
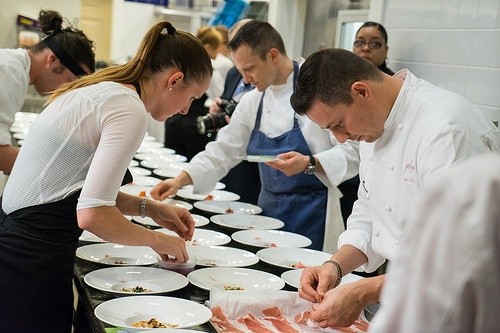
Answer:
[9,132,299,333]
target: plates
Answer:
[281,269,365,289]
[93,295,212,333]
[154,228,231,246]
[231,229,313,248]
[118,131,314,229]
[78,229,109,243]
[169,246,259,267]
[76,243,162,266]
[9,111,40,150]
[187,267,286,291]
[256,247,337,270]
[83,266,190,295]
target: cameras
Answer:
[199,98,238,134]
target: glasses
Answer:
[355,40,386,50]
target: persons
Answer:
[290,48,500,328]
[148,18,362,252]
[369,151,500,333]
[0,20,213,333]
[338,20,396,226]
[165,19,261,205]
[0,10,97,172]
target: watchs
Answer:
[306,155,316,175]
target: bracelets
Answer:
[141,197,146,218]
[322,260,342,287]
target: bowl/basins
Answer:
[156,252,198,277]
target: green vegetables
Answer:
[133,285,144,292]
[226,286,243,290]
